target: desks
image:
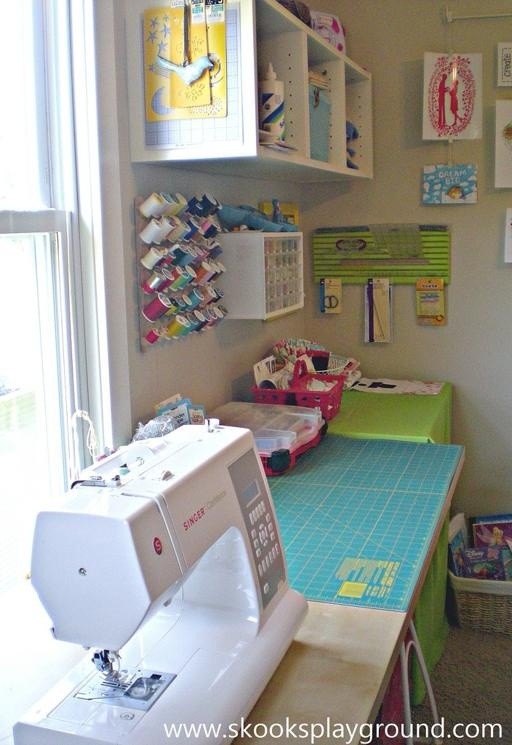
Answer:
[233,433,467,744]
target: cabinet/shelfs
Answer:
[284,374,454,709]
[222,227,307,322]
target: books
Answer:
[446,511,512,581]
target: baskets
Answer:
[251,354,349,420]
[446,568,511,638]
